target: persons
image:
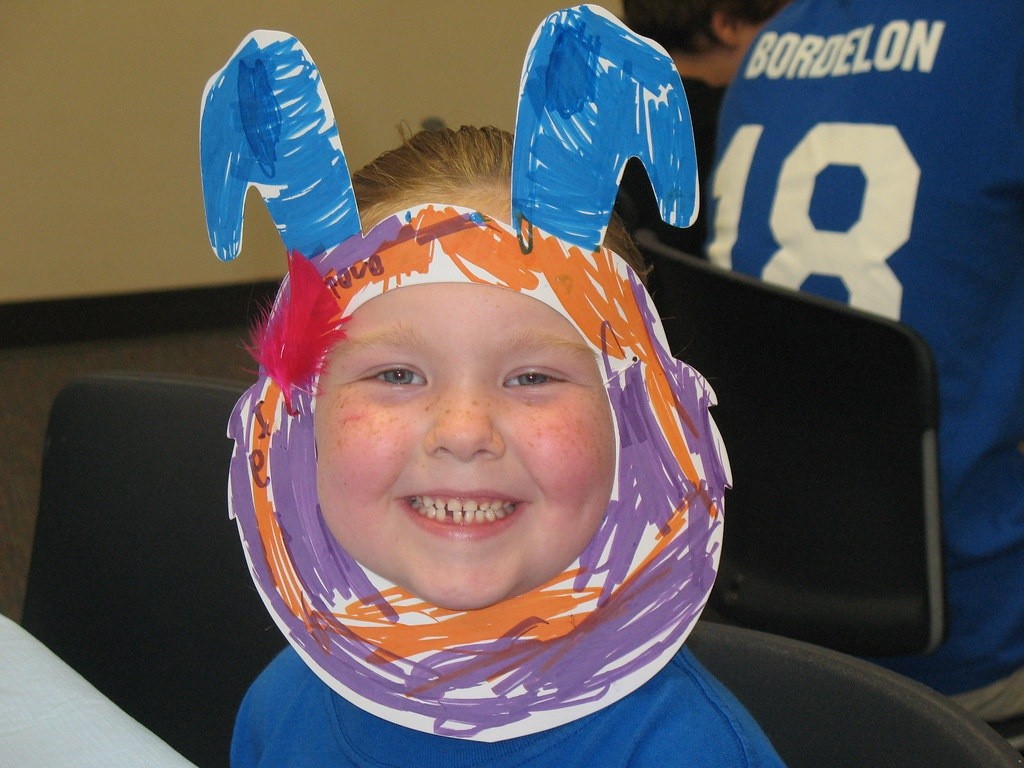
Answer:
[229,125,789,768]
[701,0,1024,724]
[615,0,787,267]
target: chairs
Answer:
[19,372,290,768]
[634,229,944,658]
[684,622,1024,767]
